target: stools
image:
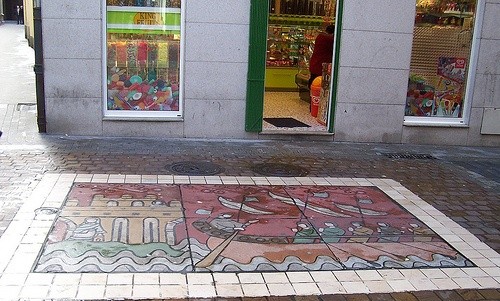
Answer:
[295,73,313,105]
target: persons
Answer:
[306,25,335,87]
[20,6,24,24]
[16,6,21,24]
